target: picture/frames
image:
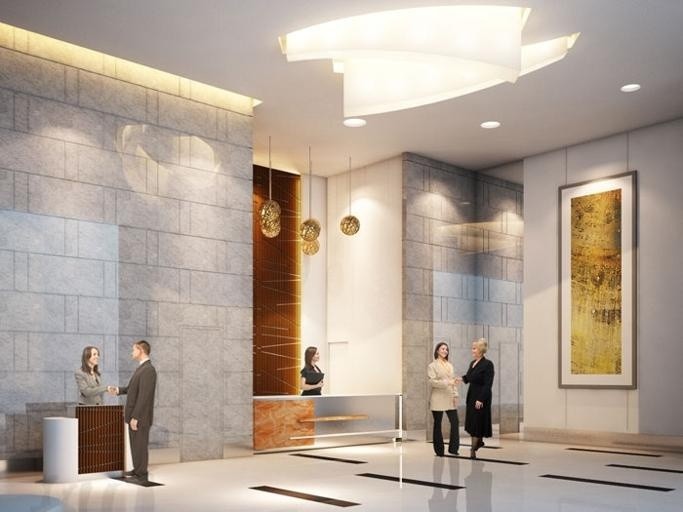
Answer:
[558,170,637,390]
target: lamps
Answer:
[259,136,360,256]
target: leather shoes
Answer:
[122,470,148,485]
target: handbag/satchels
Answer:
[306,371,324,385]
